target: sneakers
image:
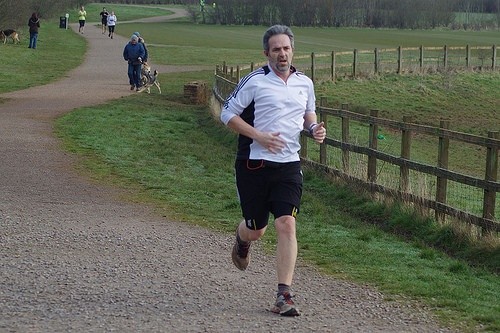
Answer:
[136,89,140,92]
[79,29,80,32]
[131,85,135,90]
[272,292,300,316]
[81,31,84,34]
[232,228,249,271]
[111,36,112,39]
[109,34,110,37]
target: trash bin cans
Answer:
[60,16,66,28]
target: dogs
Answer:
[137,66,162,94]
[0,29,20,44]
